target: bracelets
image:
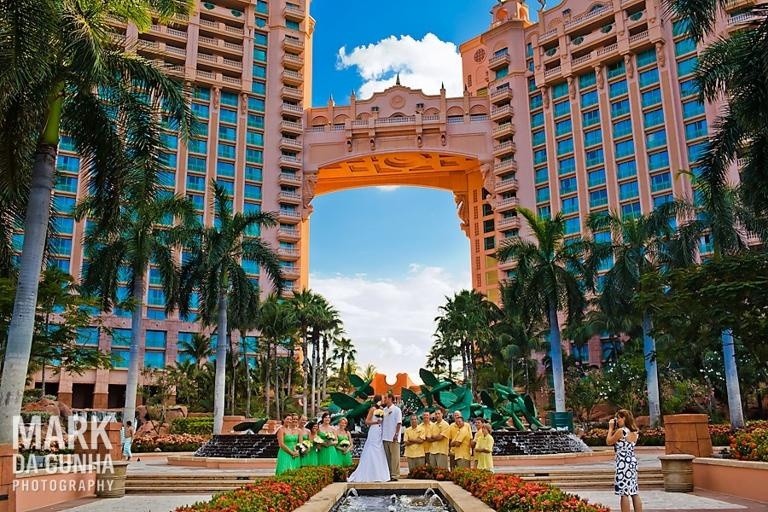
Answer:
[395,433,399,436]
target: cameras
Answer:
[613,419,618,428]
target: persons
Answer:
[347,395,391,483]
[275,413,353,477]
[402,408,494,472]
[382,394,402,482]
[117,416,125,454]
[123,421,134,461]
[606,409,643,512]
